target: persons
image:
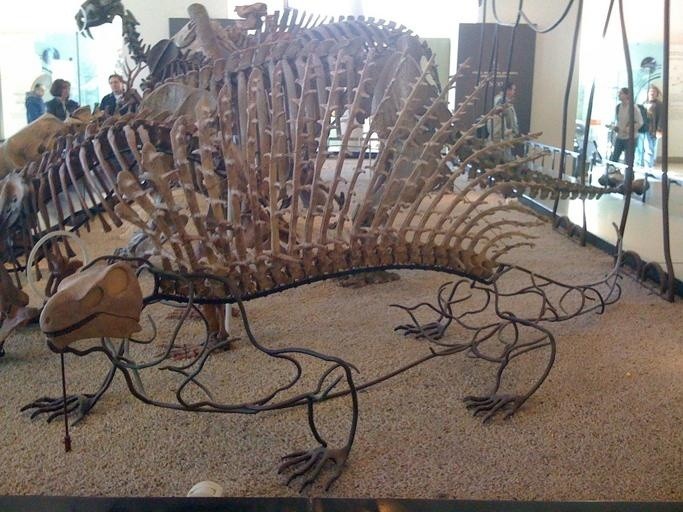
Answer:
[608,87,643,167]
[45,78,80,121]
[636,84,663,167]
[24,83,45,124]
[488,80,518,154]
[100,74,135,115]
